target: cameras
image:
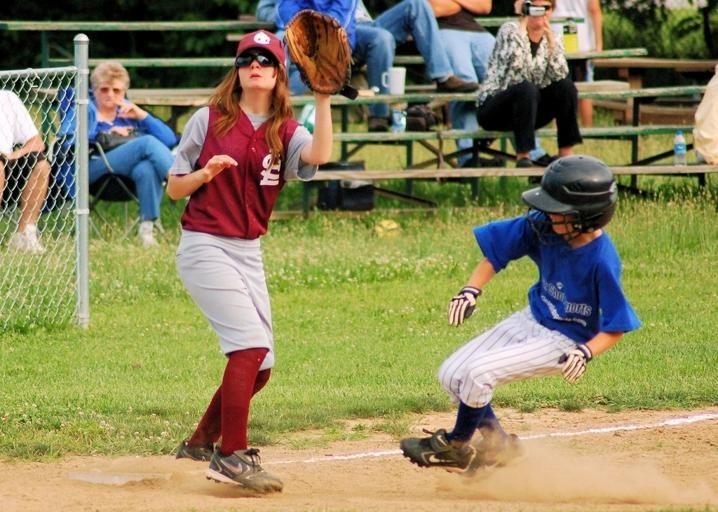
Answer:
[521,0,546,16]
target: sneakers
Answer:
[479,433,524,470]
[436,73,480,94]
[176,437,215,463]
[138,221,168,248]
[516,156,532,167]
[394,427,476,476]
[10,226,46,255]
[204,446,285,498]
[368,117,388,133]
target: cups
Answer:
[391,109,408,134]
[381,65,408,96]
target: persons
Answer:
[256,0,603,167]
[694,63,718,165]
[165,8,358,494]
[54,60,177,246]
[399,154,641,484]
[0,88,53,255]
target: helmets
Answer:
[521,155,619,233]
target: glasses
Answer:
[234,53,272,68]
[99,86,122,95]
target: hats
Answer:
[237,29,287,67]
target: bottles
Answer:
[560,16,580,53]
[672,130,687,167]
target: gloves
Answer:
[446,286,483,328]
[557,343,593,383]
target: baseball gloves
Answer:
[285,9,351,94]
